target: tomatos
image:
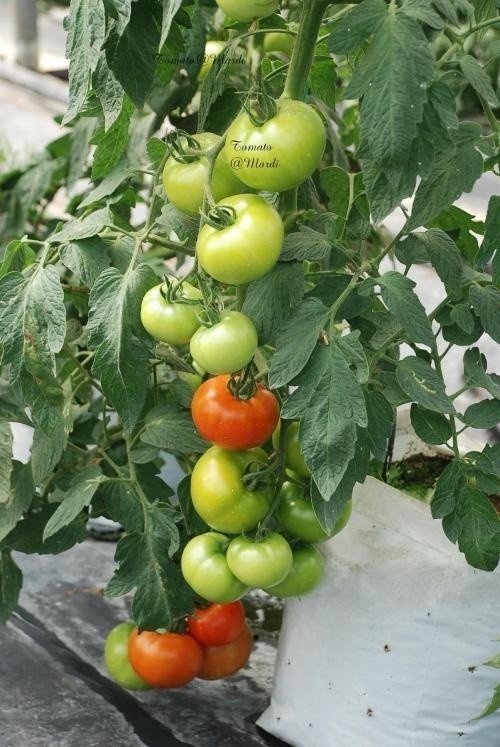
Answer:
[103,0,352,691]
